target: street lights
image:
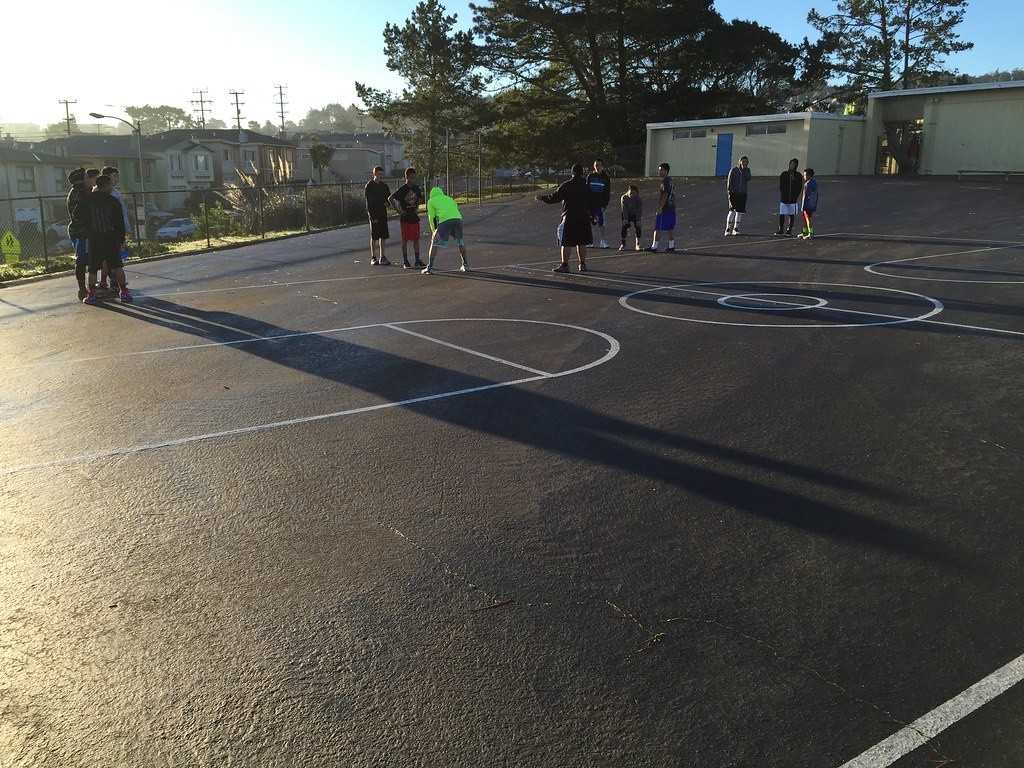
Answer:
[89,113,146,212]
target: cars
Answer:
[155,218,196,240]
[46,218,70,241]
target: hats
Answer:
[571,163,583,175]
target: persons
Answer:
[644,163,677,252]
[776,158,803,237]
[723,156,752,236]
[67,167,134,303]
[365,167,470,274]
[584,161,611,248]
[796,168,819,241]
[618,186,642,251]
[533,163,597,272]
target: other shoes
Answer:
[664,246,675,252]
[636,243,642,251]
[732,227,740,235]
[797,233,807,238]
[803,233,814,239]
[643,246,657,252]
[774,230,784,237]
[619,244,625,250]
[90,280,129,294]
[783,231,792,237]
[78,290,89,299]
[725,228,730,236]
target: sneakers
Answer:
[83,291,97,303]
[370,256,390,265]
[119,292,132,302]
[578,262,586,271]
[460,263,469,271]
[599,241,610,248]
[415,259,427,267]
[421,265,434,275]
[552,264,570,273]
[586,243,594,248]
[403,262,412,268]
